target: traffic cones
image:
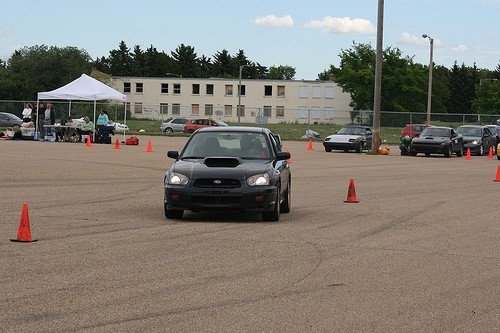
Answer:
[111,136,122,150]
[487,146,495,158]
[464,148,472,160]
[492,162,500,182]
[8,200,39,243]
[285,148,293,164]
[306,137,314,150]
[144,138,156,152]
[83,133,94,147]
[342,176,360,204]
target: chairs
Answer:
[240,135,266,159]
[203,137,228,156]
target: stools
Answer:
[81,135,88,142]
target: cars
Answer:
[159,117,190,134]
[410,125,464,158]
[161,124,293,222]
[0,112,23,128]
[322,125,383,154]
[455,124,496,157]
[484,124,498,139]
[105,120,130,132]
[182,117,229,134]
[496,126,500,160]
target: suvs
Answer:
[399,123,432,143]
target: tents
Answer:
[36,74,126,142]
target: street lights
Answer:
[478,78,498,123]
[238,64,251,123]
[165,72,182,78]
[421,33,434,125]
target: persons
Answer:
[78,117,94,142]
[399,135,412,156]
[22,103,33,122]
[97,109,108,130]
[38,103,46,129]
[32,103,37,132]
[45,104,56,125]
[495,119,500,154]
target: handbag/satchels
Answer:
[126,136,139,146]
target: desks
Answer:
[44,125,83,142]
